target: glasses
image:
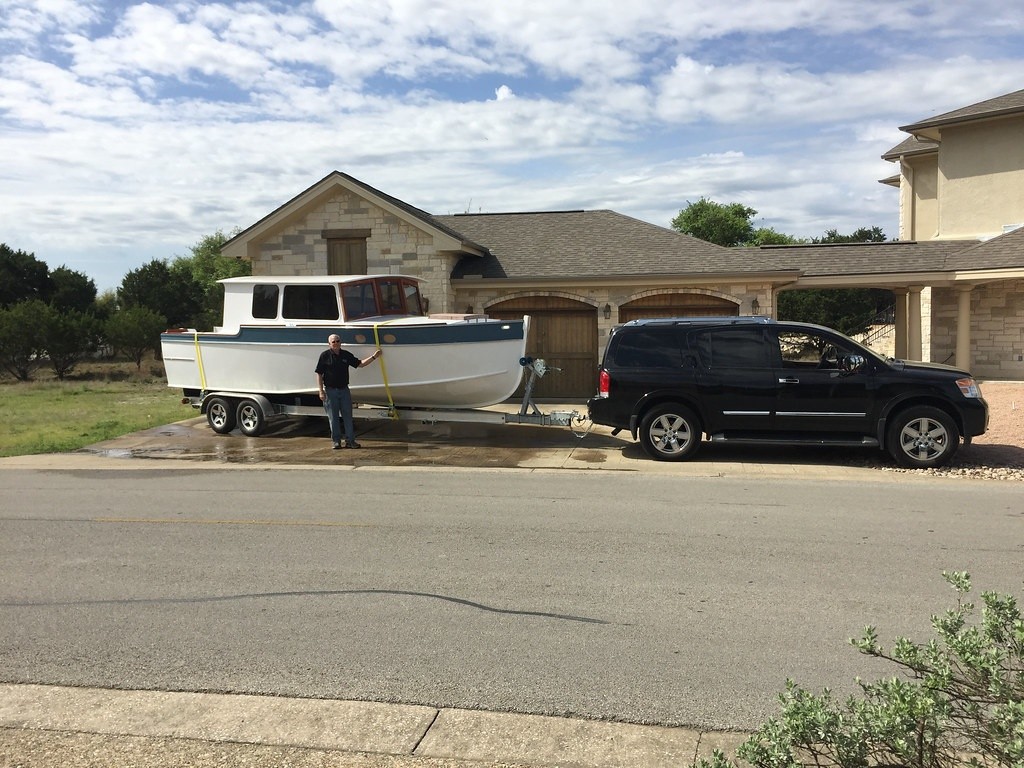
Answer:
[330,339,340,344]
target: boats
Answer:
[160,274,531,408]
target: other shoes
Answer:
[345,439,360,449]
[332,441,341,449]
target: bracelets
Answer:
[372,355,377,360]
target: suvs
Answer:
[587,316,990,469]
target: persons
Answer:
[315,334,382,449]
